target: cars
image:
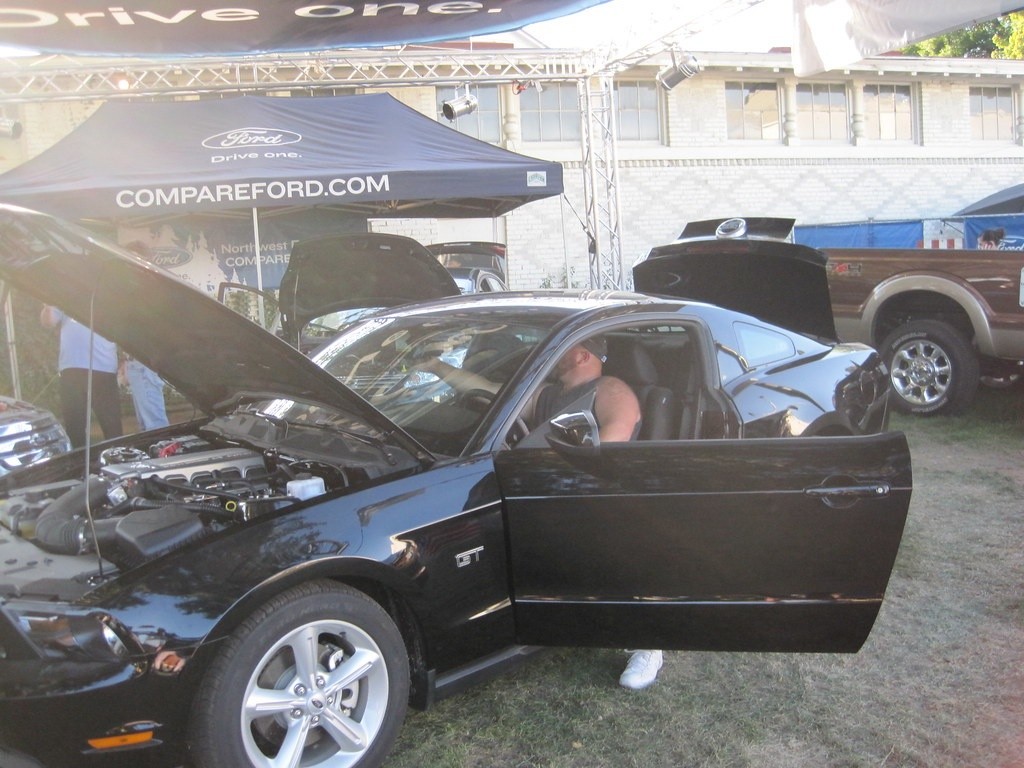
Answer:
[0,392,76,479]
[0,200,914,768]
[276,232,507,401]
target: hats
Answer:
[582,335,608,364]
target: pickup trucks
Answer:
[817,246,1024,419]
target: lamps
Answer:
[439,82,479,121]
[655,45,703,94]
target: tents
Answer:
[0,94,571,333]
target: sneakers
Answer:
[619,649,662,690]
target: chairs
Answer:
[455,331,527,401]
[607,341,676,443]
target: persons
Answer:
[976,228,1006,250]
[40,303,123,449]
[408,330,663,692]
[117,242,169,433]
